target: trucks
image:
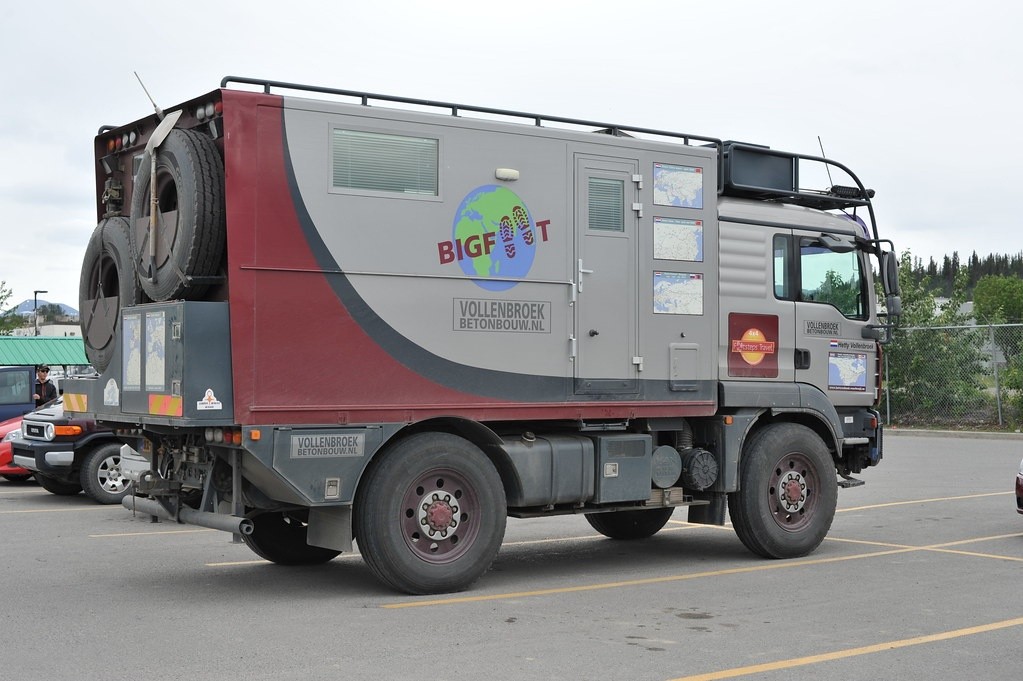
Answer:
[75,75,901,589]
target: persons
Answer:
[33,364,57,408]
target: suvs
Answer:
[11,386,141,506]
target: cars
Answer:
[0,416,39,483]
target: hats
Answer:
[38,366,50,371]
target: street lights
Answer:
[33,290,48,337]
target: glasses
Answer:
[41,369,48,373]
